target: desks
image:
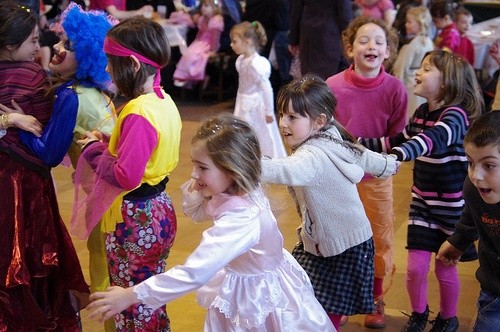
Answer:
[466,15,500,69]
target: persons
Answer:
[86,116,338,332]
[0,0,500,332]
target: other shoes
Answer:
[339,315,348,327]
[365,300,386,328]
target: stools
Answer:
[182,51,229,102]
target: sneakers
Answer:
[402,304,429,332]
[429,312,459,332]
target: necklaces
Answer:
[90,85,164,136]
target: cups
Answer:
[157,5,167,19]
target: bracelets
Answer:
[0,113,14,130]
